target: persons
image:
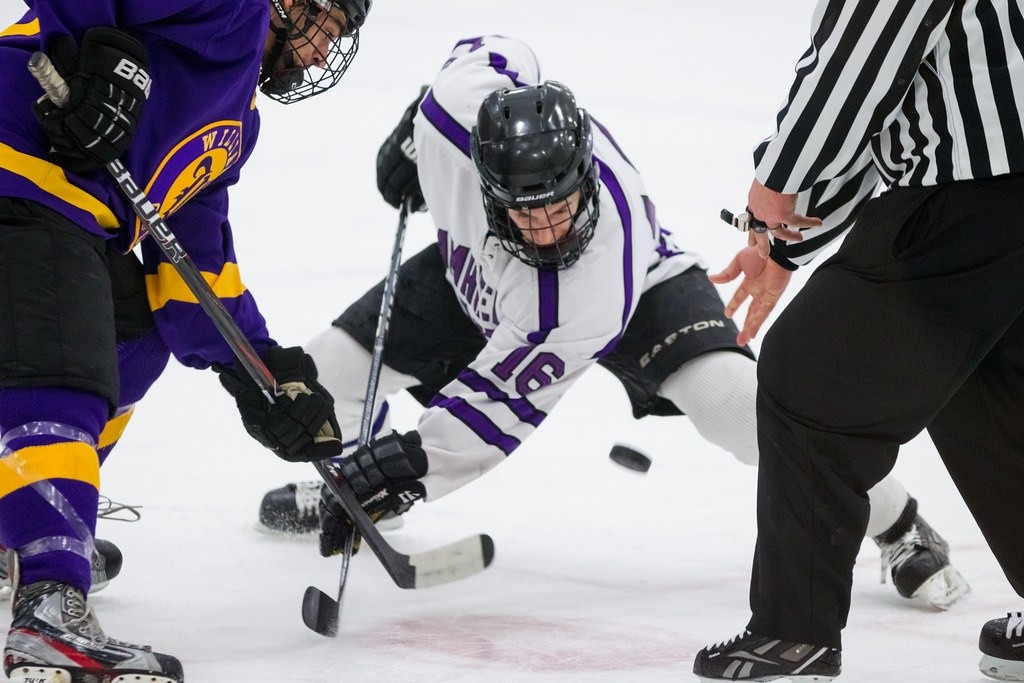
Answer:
[0,0,375,683]
[256,34,970,609]
[692,0,1024,683]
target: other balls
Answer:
[607,441,655,475]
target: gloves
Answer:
[36,24,156,173]
[376,85,426,214]
[211,344,344,463]
[313,431,430,557]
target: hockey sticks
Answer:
[300,192,413,639]
[26,50,495,590]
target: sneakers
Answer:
[977,610,1024,682]
[2,580,186,682]
[872,494,972,615]
[259,477,428,536]
[692,627,843,682]
[0,538,125,597]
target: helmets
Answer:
[257,0,374,107]
[468,80,602,272]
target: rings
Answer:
[768,225,781,233]
